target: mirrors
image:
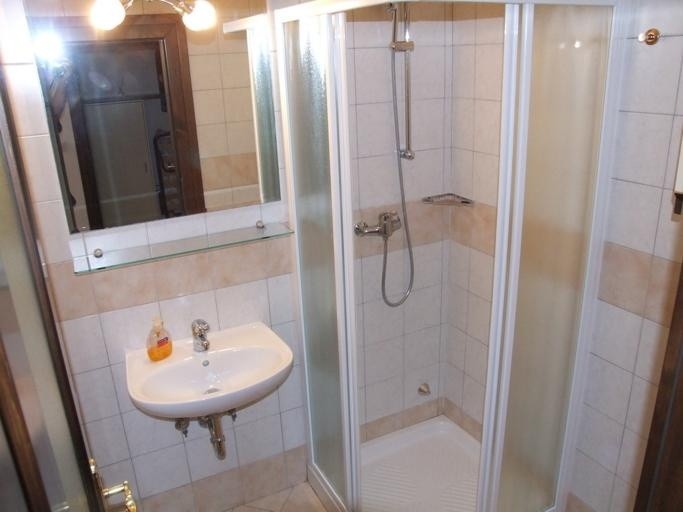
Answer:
[22,0,282,236]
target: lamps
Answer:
[86,0,218,32]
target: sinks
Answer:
[125,320,293,420]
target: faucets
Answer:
[190,319,210,353]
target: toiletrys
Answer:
[146,314,172,362]
